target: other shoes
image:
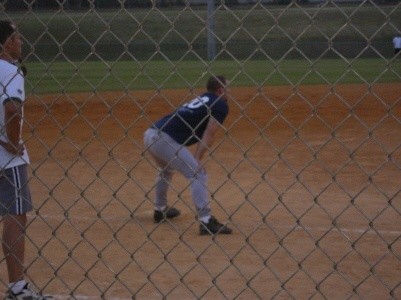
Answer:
[153,205,180,222]
[199,215,232,235]
[8,282,55,300]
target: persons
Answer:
[143,75,230,235]
[0,20,46,300]
[393,31,401,59]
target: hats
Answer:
[0,20,17,51]
